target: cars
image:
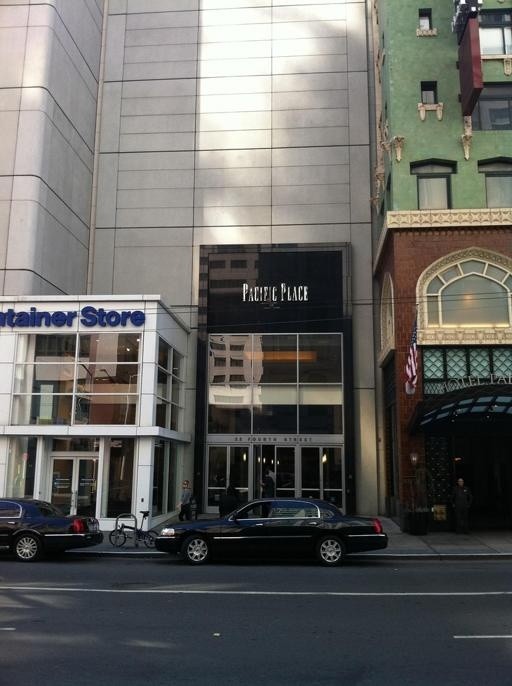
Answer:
[0,496,104,563]
[152,496,388,565]
[51,475,117,507]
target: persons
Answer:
[260,471,275,518]
[178,479,195,522]
[451,477,474,536]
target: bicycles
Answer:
[110,511,159,549]
